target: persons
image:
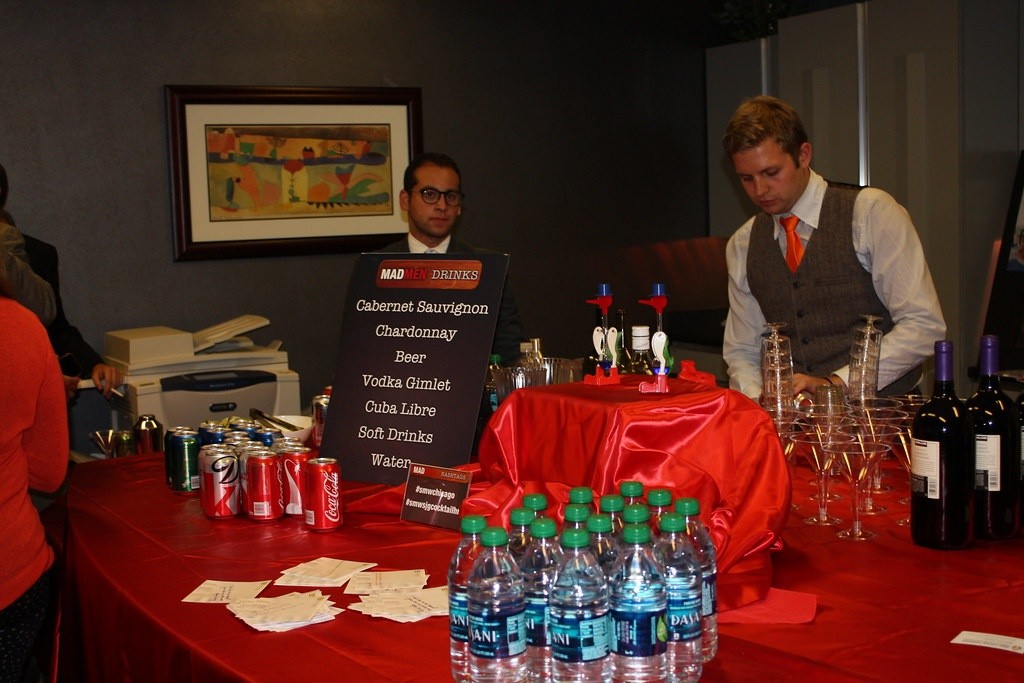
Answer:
[373,151,528,457]
[719,95,947,457]
[0,169,123,683]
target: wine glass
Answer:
[761,312,883,405]
[764,393,933,540]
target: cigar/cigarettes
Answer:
[110,388,123,397]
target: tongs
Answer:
[249,407,304,434]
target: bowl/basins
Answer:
[257,415,313,443]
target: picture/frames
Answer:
[164,84,425,263]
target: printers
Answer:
[103,314,300,450]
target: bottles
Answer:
[447,481,718,683]
[116,415,164,456]
[1017,392,1024,527]
[488,338,542,368]
[581,308,654,376]
[910,340,971,549]
[966,334,1019,541]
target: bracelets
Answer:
[824,377,834,385]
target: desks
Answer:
[45,434,1024,683]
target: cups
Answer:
[478,357,583,434]
[89,428,121,458]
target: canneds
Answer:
[164,418,343,531]
[312,386,332,445]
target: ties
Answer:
[779,215,806,278]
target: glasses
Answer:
[410,186,466,207]
